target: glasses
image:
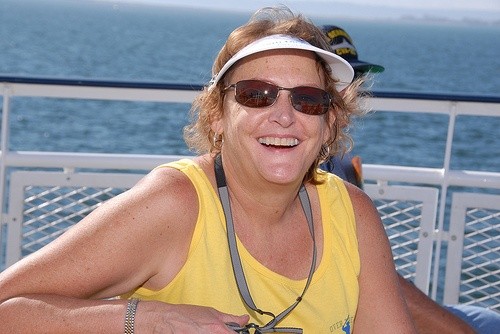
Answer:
[220,79,331,115]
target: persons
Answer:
[0,4,418,334]
[316,24,500,334]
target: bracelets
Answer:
[124,298,140,334]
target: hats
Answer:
[318,24,385,74]
[215,34,355,92]
